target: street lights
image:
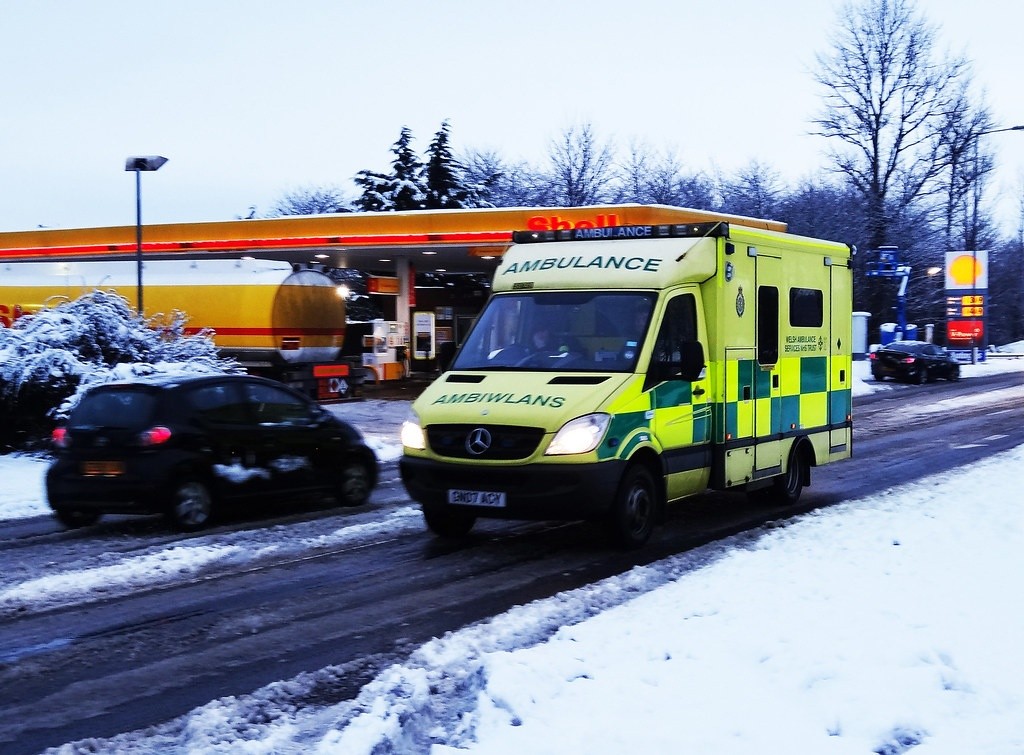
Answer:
[970,124,1024,365]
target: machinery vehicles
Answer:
[0,256,346,365]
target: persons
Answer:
[616,303,681,362]
[524,316,570,352]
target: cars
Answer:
[43,371,380,528]
[870,340,959,386]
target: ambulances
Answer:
[399,219,854,546]
